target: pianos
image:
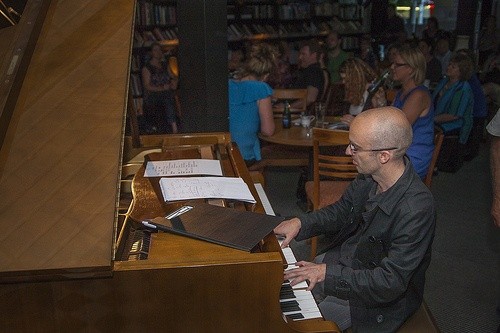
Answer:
[1,0,339,333]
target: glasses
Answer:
[394,62,408,68]
[350,142,397,153]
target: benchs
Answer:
[346,297,441,333]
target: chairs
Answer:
[304,127,365,261]
[262,88,310,166]
[307,70,328,115]
[423,130,444,190]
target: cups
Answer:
[305,125,312,139]
[312,102,326,128]
[424,79,429,87]
[389,80,394,89]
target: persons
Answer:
[485,105,500,227]
[375,4,407,42]
[227,16,500,215]
[273,107,436,333]
[142,43,178,134]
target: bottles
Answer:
[282,100,291,129]
[367,71,391,95]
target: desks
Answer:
[259,116,351,214]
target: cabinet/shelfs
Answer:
[126,0,373,120]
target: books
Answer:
[129,0,181,123]
[227,0,372,51]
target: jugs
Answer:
[300,115,315,127]
